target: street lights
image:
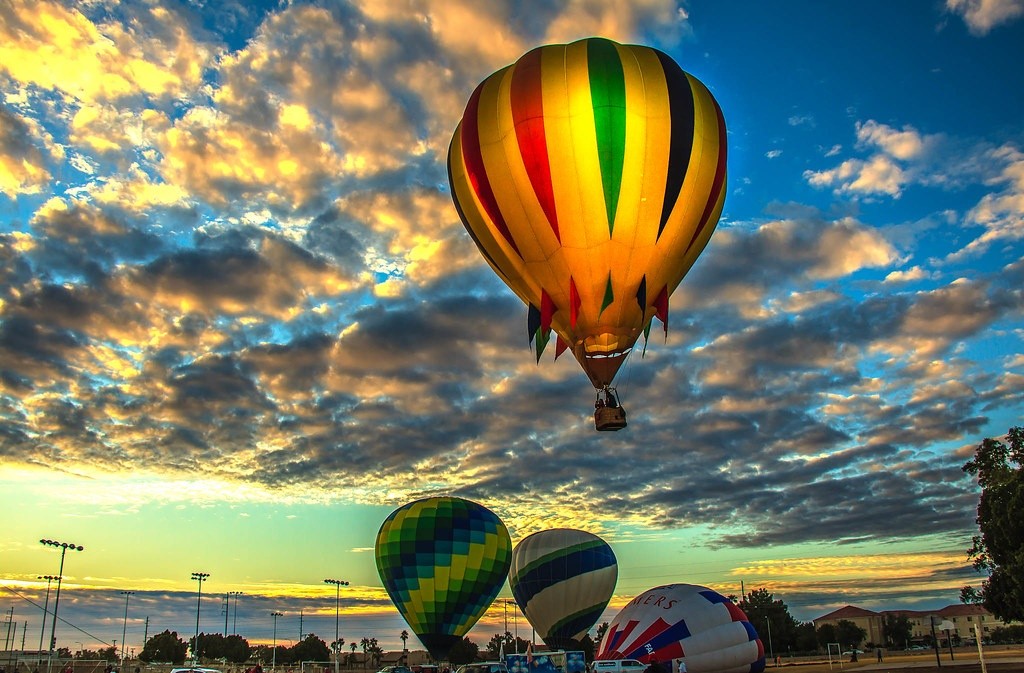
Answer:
[270,612,283,668]
[75,642,83,655]
[324,579,350,673]
[229,591,243,635]
[190,572,210,659]
[112,640,118,647]
[130,648,136,660]
[37,539,85,658]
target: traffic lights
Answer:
[119,591,136,666]
[52,638,56,648]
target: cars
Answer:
[170,667,222,673]
[376,666,412,673]
[410,665,439,673]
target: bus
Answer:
[302,661,339,673]
[505,651,586,673]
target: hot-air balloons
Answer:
[508,528,619,653]
[446,38,727,432]
[593,584,766,673]
[375,495,513,662]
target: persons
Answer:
[877,649,883,662]
[853,650,858,663]
[60,666,73,673]
[104,664,120,673]
[227,666,251,673]
[442,667,450,673]
[595,399,606,408]
[675,659,688,673]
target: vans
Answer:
[590,659,648,673]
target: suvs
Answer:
[457,663,509,673]
